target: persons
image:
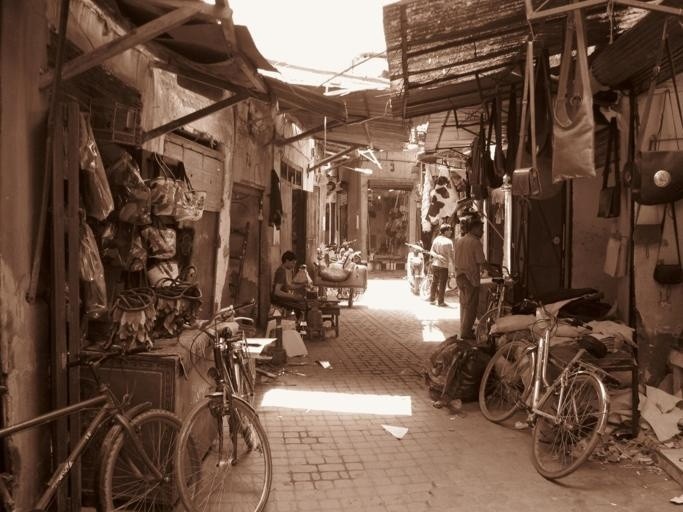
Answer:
[340,241,355,262]
[425,222,454,306]
[270,250,307,320]
[453,218,504,339]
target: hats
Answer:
[468,219,484,230]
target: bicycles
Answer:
[419,259,434,301]
[171,294,274,512]
[474,261,520,353]
[478,296,622,480]
[0,342,202,512]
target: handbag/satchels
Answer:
[106,265,204,357]
[146,259,179,289]
[599,186,621,219]
[174,161,207,223]
[493,147,506,177]
[632,222,663,247]
[550,97,598,185]
[141,217,178,260]
[77,111,152,321]
[148,150,187,218]
[602,228,630,280]
[622,162,638,189]
[484,150,503,189]
[634,148,683,208]
[653,263,683,286]
[512,165,543,198]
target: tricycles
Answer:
[313,238,368,309]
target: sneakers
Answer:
[460,329,477,340]
[430,299,449,308]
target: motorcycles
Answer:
[404,239,430,295]
